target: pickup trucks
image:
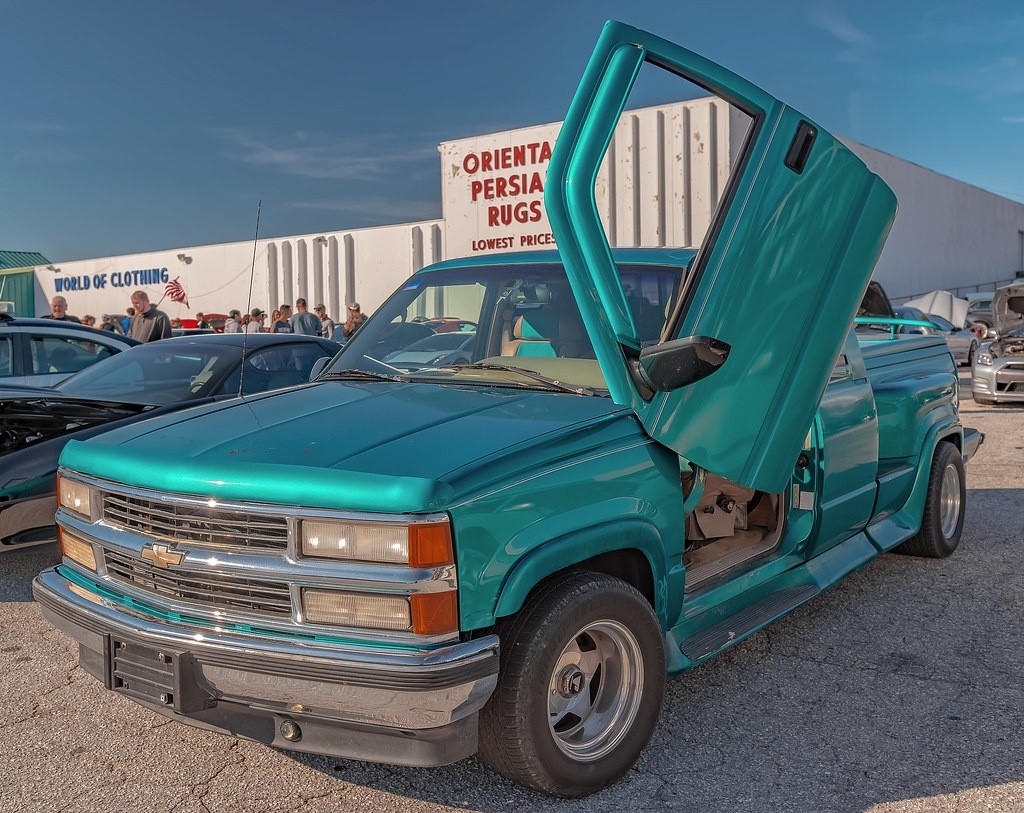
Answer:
[31,18,986,799]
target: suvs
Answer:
[0,310,202,395]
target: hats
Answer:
[348,302,360,310]
[228,309,240,318]
[252,308,264,317]
[314,303,325,309]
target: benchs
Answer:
[47,347,112,374]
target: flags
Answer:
[165,280,190,309]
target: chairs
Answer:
[679,468,755,541]
[499,310,583,361]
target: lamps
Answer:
[46,264,61,273]
[177,253,192,264]
[315,236,328,246]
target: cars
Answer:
[331,315,478,360]
[971,282,1024,405]
[386,331,476,374]
[855,282,993,367]
[0,332,366,555]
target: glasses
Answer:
[316,309,320,311]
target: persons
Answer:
[288,298,323,370]
[98,307,135,335]
[195,312,206,328]
[126,290,173,343]
[342,302,368,342]
[269,305,293,370]
[71,314,96,352]
[241,308,264,332]
[223,309,243,333]
[266,310,281,333]
[173,318,184,328]
[313,303,334,338]
[39,295,82,353]
